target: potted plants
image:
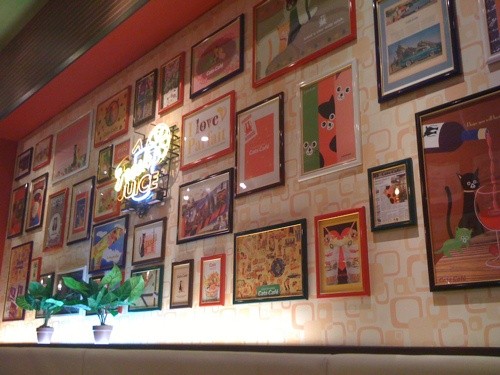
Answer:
[15,280,91,345]
[45,265,147,345]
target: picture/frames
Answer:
[2,0,500,322]
